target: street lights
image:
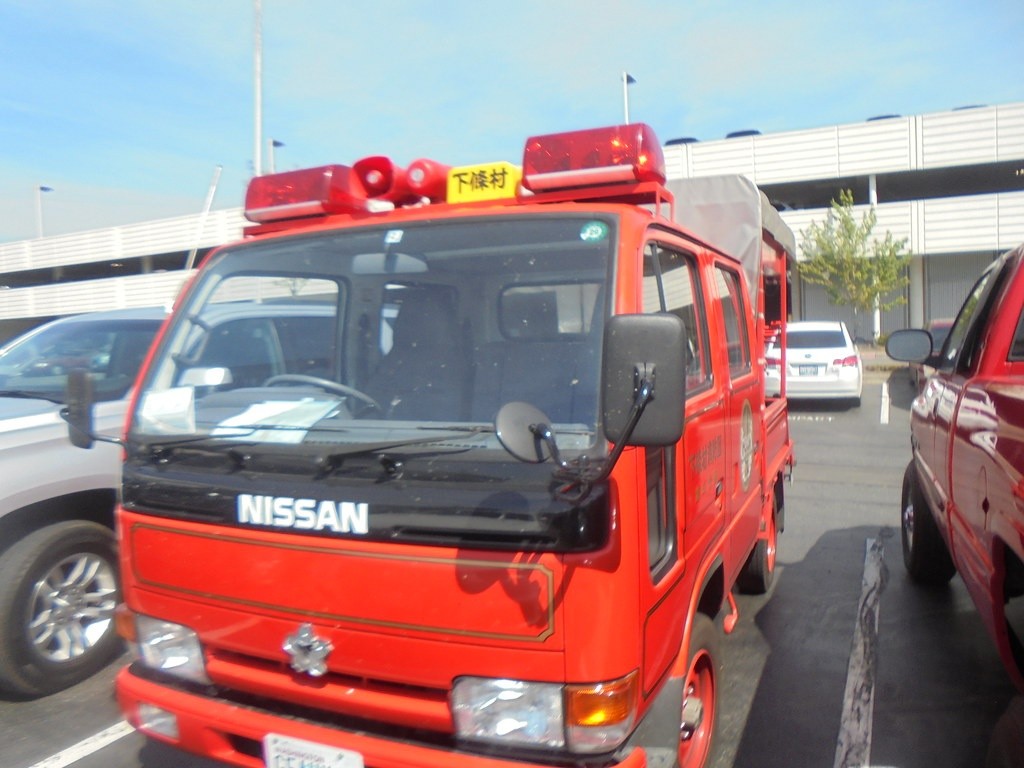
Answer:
[622,71,638,125]
[266,138,284,173]
[36,185,55,238]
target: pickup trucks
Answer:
[883,243,1023,699]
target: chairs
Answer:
[199,333,272,391]
[462,287,560,346]
[106,328,159,379]
[468,336,597,429]
[363,281,471,423]
[589,277,658,336]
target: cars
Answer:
[762,321,867,408]
[0,302,400,700]
[907,317,956,391]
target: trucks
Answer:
[106,121,796,768]
